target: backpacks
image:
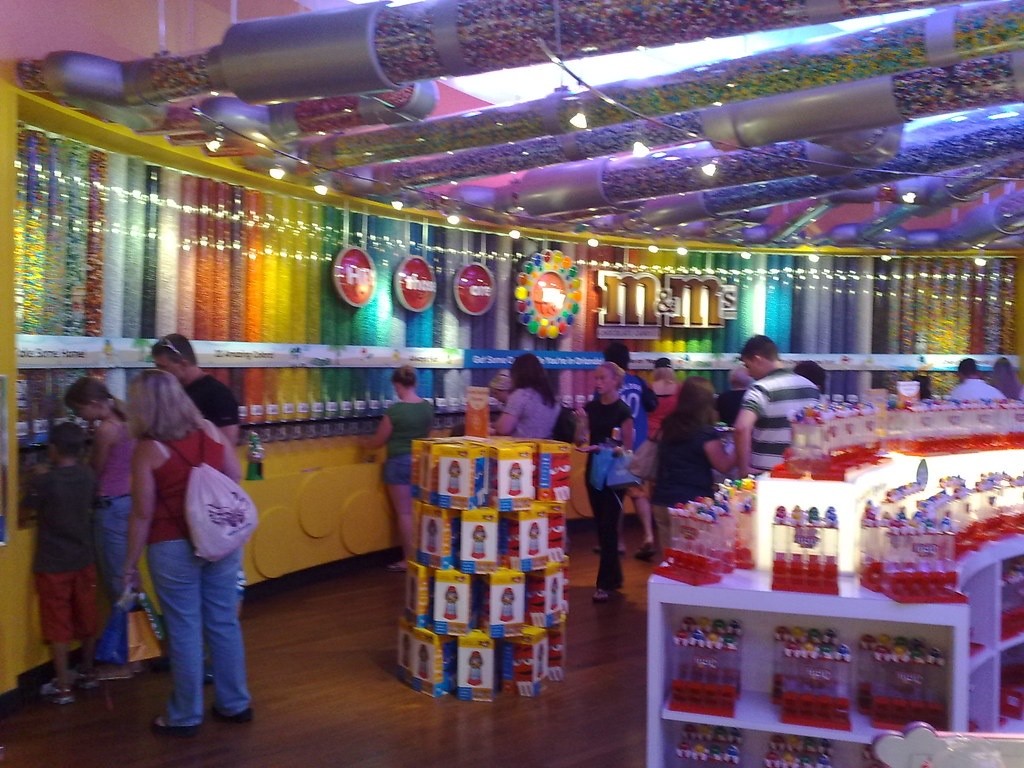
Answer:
[140,427,257,563]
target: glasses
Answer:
[156,336,185,359]
[713,393,719,399]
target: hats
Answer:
[726,366,754,391]
[488,369,513,390]
[655,358,672,368]
[653,368,675,395]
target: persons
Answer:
[989,356,1024,399]
[650,375,740,559]
[591,343,658,559]
[488,352,562,441]
[713,366,754,426]
[121,369,254,741]
[793,360,826,394]
[951,359,1007,399]
[66,377,146,681]
[17,423,101,705]
[733,335,823,477]
[152,332,246,680]
[571,362,636,604]
[647,359,683,444]
[359,365,435,572]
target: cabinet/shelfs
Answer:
[646,452,1024,768]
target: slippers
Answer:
[592,543,626,556]
[634,546,657,560]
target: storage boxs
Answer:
[397,434,574,702]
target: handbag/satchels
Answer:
[553,401,578,445]
[96,582,165,665]
[628,427,661,480]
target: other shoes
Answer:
[211,704,255,724]
[152,717,198,737]
[130,662,143,673]
[65,668,99,690]
[386,561,408,572]
[591,588,613,603]
[39,682,74,705]
[96,665,132,680]
